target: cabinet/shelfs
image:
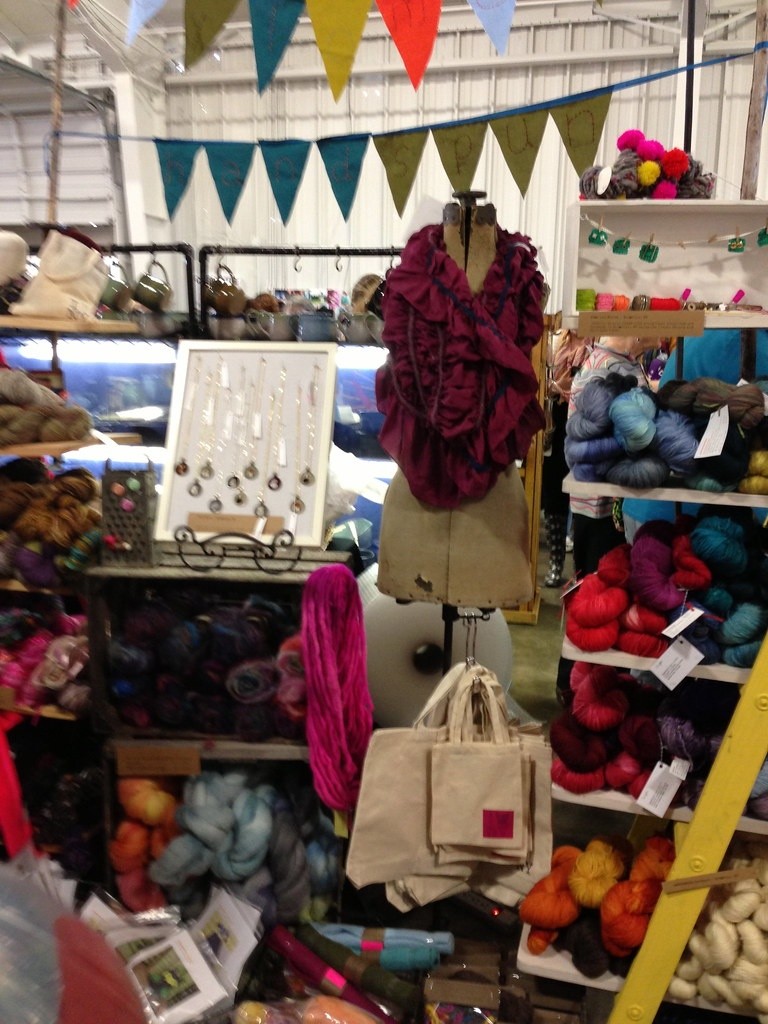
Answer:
[2,199,768,1024]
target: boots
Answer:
[543,511,568,587]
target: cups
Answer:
[101,261,132,312]
[298,312,338,342]
[337,312,372,344]
[209,263,246,316]
[133,261,174,315]
[363,310,387,346]
[260,312,296,340]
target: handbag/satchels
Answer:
[345,661,554,914]
